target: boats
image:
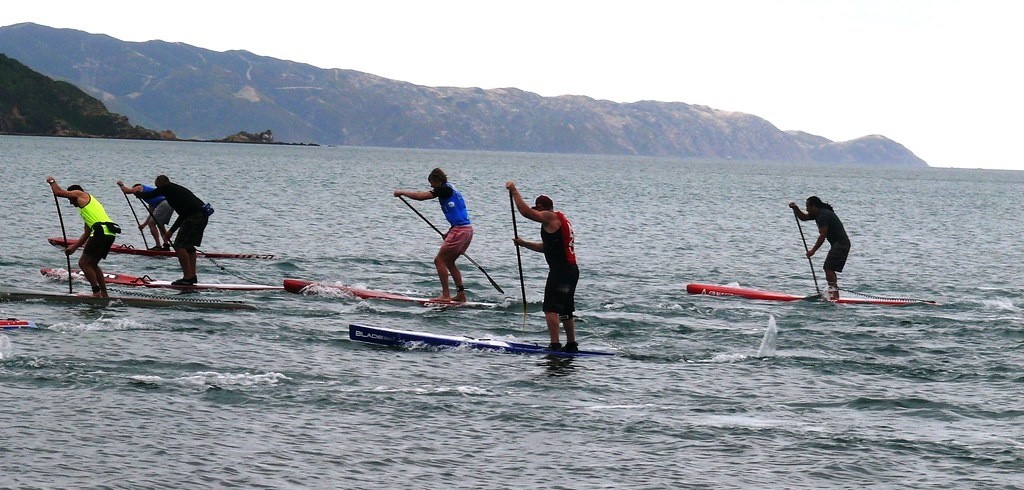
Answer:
[48,235,279,262]
[346,322,616,360]
[42,264,286,292]
[280,277,502,311]
[686,282,928,307]
[1,317,41,333]
[0,285,263,311]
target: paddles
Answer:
[394,190,507,298]
[47,178,76,298]
[117,182,180,262]
[504,181,533,304]
[791,200,826,302]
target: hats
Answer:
[531,195,553,211]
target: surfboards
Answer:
[279,271,500,320]
[1,318,75,337]
[2,233,285,311]
[684,279,938,306]
[346,322,613,363]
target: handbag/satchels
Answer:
[105,221,121,234]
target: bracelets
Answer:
[50,179,55,184]
[120,184,124,186]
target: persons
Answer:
[506,181,580,353]
[117,180,174,250]
[134,175,209,285]
[789,196,852,299]
[48,177,116,297]
[394,168,473,301]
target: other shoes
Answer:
[148,245,170,251]
[170,275,197,286]
[544,341,579,354]
